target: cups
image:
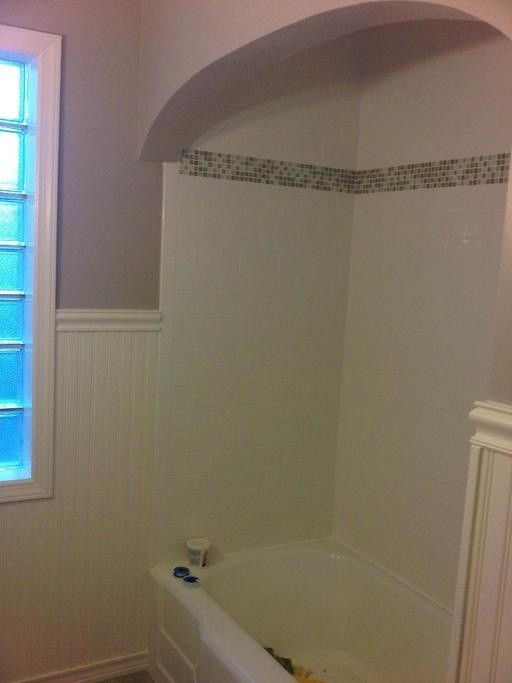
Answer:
[186,538,211,570]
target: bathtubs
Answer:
[147,537,455,683]
